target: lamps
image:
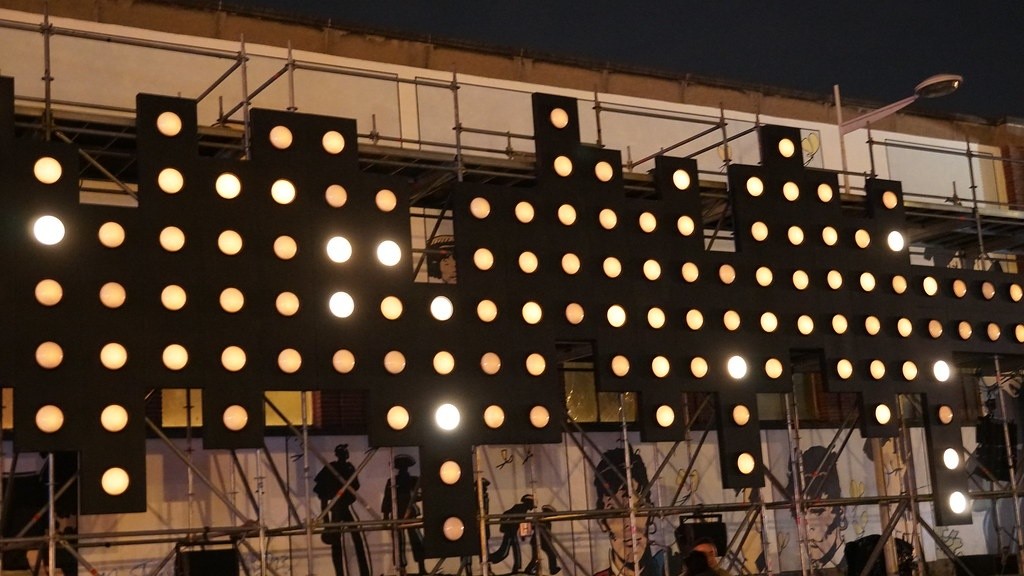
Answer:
[4,75,1024,550]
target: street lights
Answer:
[833,73,963,197]
